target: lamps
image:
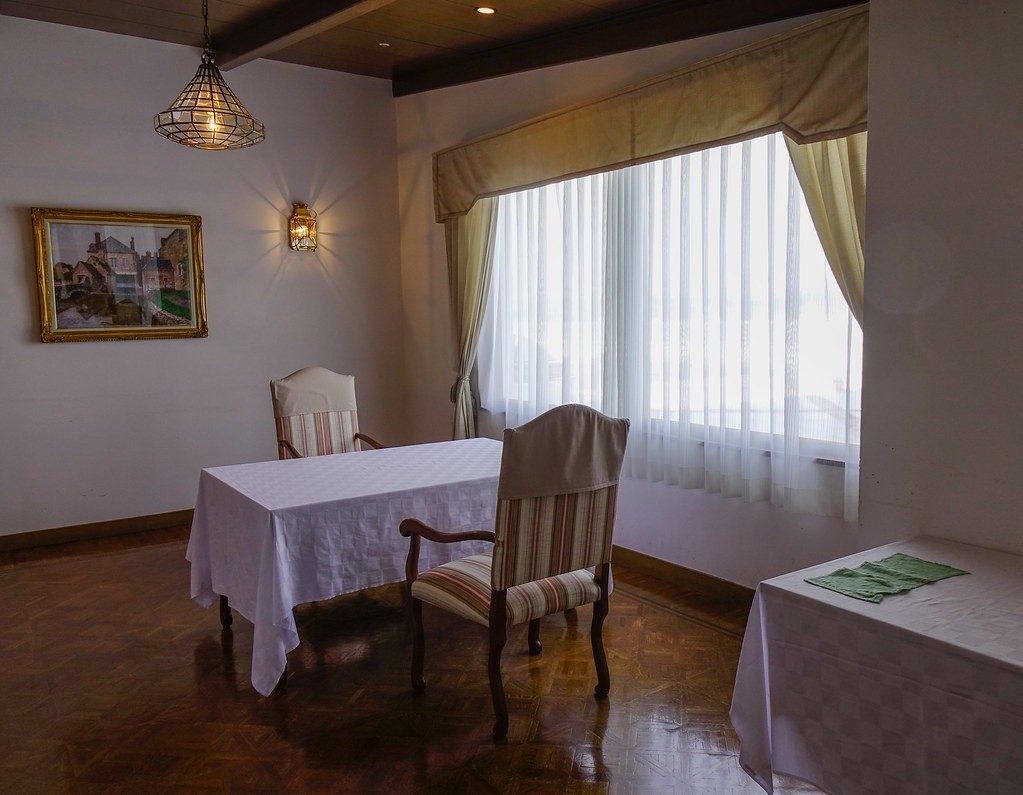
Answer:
[288,202,318,253]
[152,0,266,151]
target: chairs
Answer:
[269,366,386,459]
[397,404,631,743]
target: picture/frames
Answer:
[30,206,209,343]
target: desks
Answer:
[185,436,613,699]
[726,534,1023,795]
